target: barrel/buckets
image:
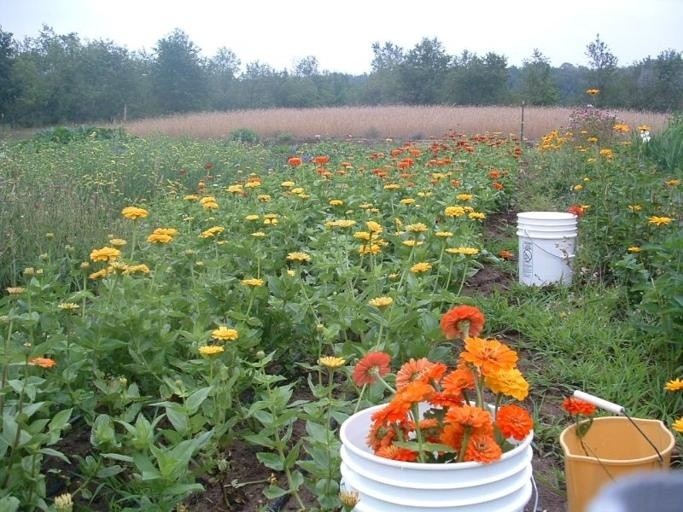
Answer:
[338,400,539,512]
[559,390,676,511]
[516,211,578,289]
